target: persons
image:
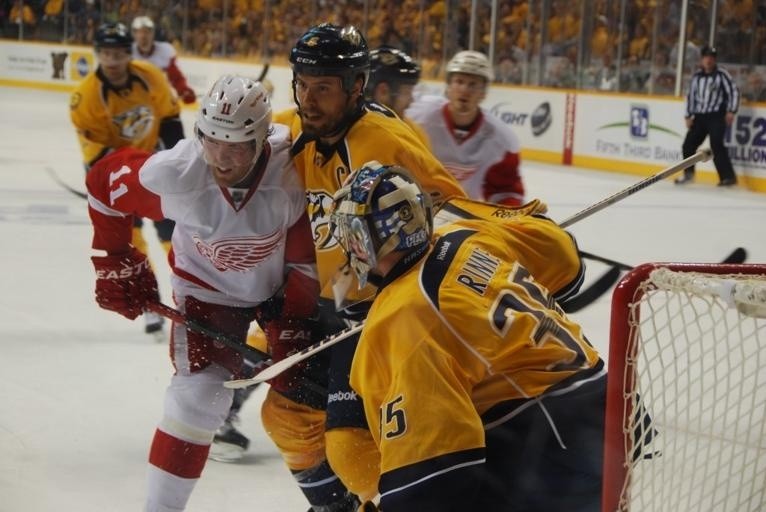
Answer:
[474,1,765,101]
[258,21,470,510]
[673,46,742,187]
[68,22,185,330]
[410,49,522,208]
[84,74,321,511]
[225,1,476,72]
[1,1,227,57]
[363,44,434,155]
[326,159,663,509]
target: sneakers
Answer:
[675,174,693,183]
[718,178,736,185]
[208,417,248,459]
[143,313,165,333]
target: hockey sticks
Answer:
[579,248,747,294]
[222,148,713,390]
[47,61,270,205]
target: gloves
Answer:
[90,248,160,318]
[264,319,319,394]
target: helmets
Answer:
[365,47,420,93]
[197,74,273,160]
[446,50,493,82]
[328,161,434,291]
[130,15,155,39]
[290,23,370,95]
[92,22,133,55]
[700,47,718,56]
